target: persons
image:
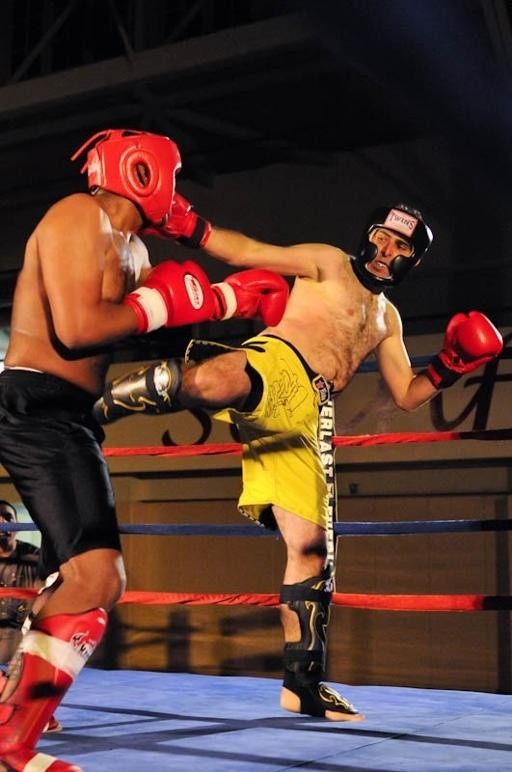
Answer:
[1,125,296,772]
[0,500,41,674]
[92,190,506,721]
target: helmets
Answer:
[356,193,437,294]
[85,123,186,231]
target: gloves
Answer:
[211,264,293,330]
[141,190,214,252]
[122,256,223,340]
[426,308,506,392]
